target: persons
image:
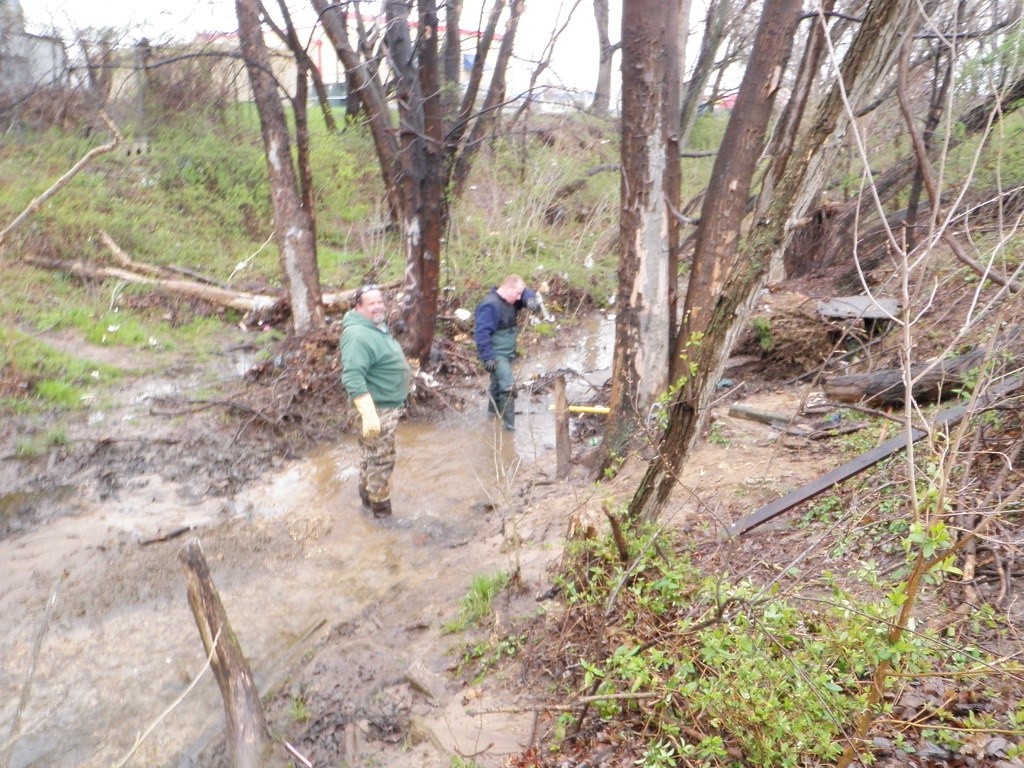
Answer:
[337,283,413,517]
[476,273,543,430]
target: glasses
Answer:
[355,284,378,298]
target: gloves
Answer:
[528,298,541,314]
[486,359,497,372]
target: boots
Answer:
[360,495,370,510]
[371,497,392,521]
